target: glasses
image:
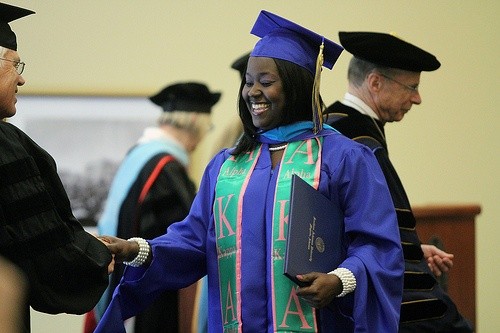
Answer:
[0,57,26,76]
[375,71,421,95]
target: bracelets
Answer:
[123,237,150,268]
[327,267,357,298]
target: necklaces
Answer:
[269,144,288,151]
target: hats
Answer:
[149,83,222,115]
[0,2,36,52]
[338,29,442,73]
[249,9,345,135]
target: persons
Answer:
[315,32,474,333]
[84,82,222,333]
[97,10,405,333]
[0,3,115,333]
